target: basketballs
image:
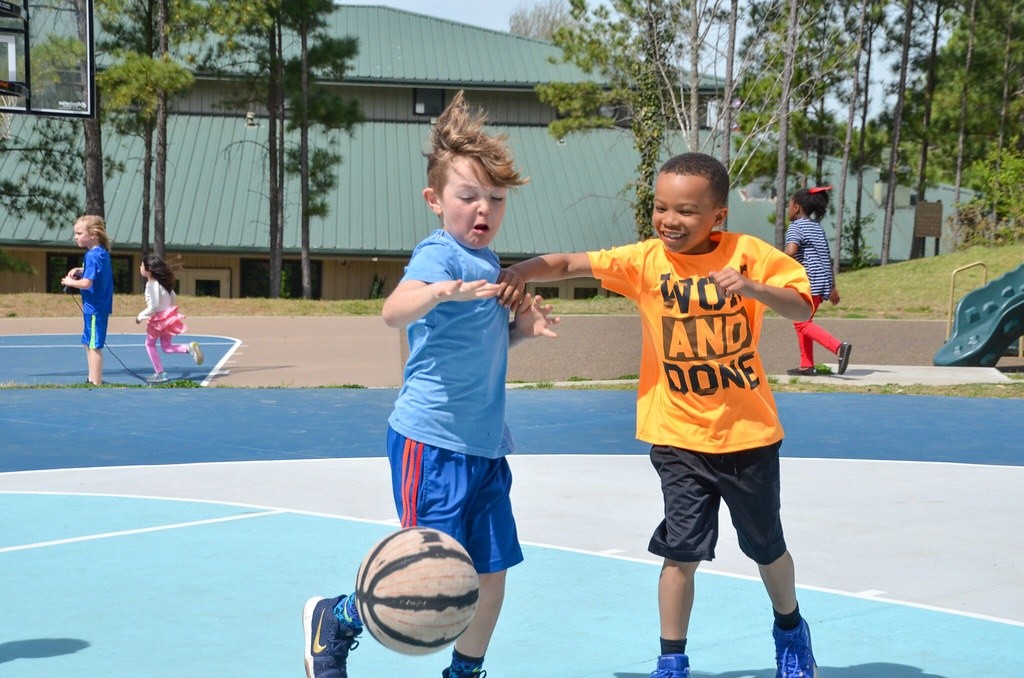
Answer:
[353,524,482,658]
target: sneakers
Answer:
[838,343,852,374]
[189,341,203,365]
[303,595,362,678]
[772,617,818,678]
[147,372,168,382]
[787,367,816,376]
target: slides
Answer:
[930,257,1024,368]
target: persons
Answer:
[497,151,822,675]
[136,254,203,381]
[304,86,560,675]
[61,214,114,384]
[780,186,852,376]
